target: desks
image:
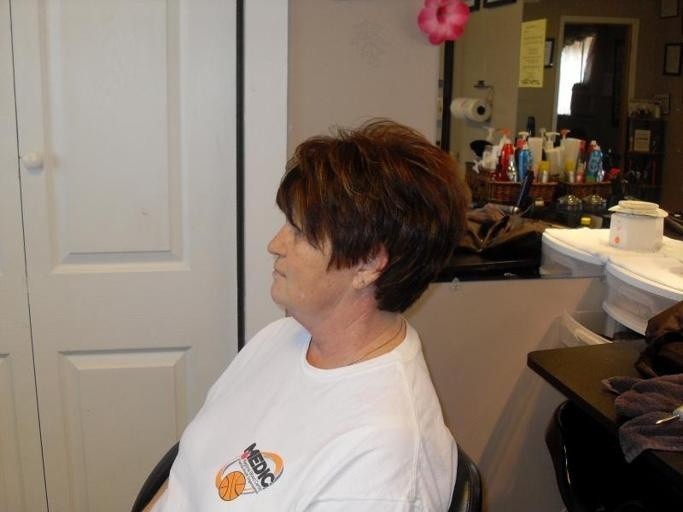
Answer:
[525,331,683,511]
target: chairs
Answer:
[127,442,483,511]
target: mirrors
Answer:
[427,0,682,281]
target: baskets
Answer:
[465,162,628,204]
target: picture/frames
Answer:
[543,38,554,67]
[663,42,683,76]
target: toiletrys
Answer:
[479,122,609,184]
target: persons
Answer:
[140,117,481,512]
